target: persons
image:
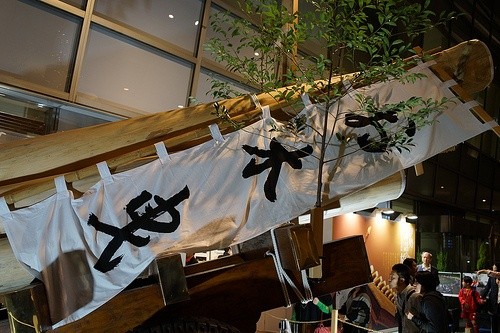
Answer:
[333,284,373,333]
[475,263,500,333]
[403,258,417,285]
[404,270,450,333]
[416,252,440,287]
[291,294,333,333]
[458,276,486,333]
[187,247,232,265]
[389,263,422,333]
